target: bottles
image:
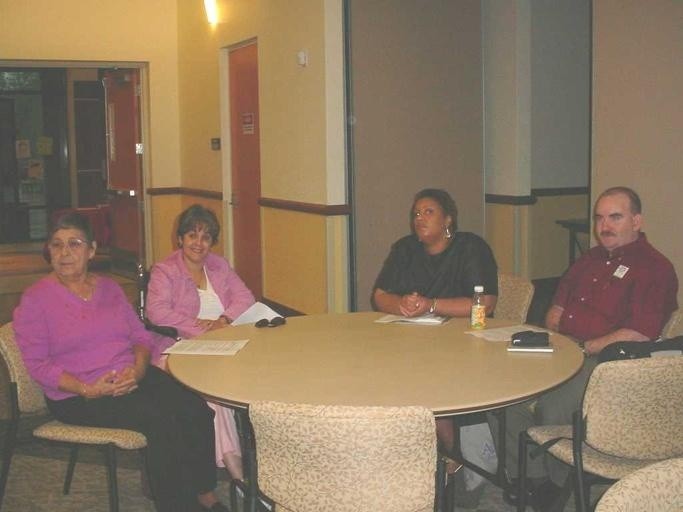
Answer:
[469,286,487,330]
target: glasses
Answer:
[255,316,286,329]
[50,238,85,253]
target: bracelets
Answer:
[576,339,587,357]
[429,296,437,313]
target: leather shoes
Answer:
[533,481,570,511]
[504,478,533,504]
[195,495,228,512]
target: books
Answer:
[371,311,452,326]
[505,337,553,353]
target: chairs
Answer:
[141,263,683,511]
[2,319,155,508]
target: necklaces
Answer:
[192,267,204,289]
[60,275,91,302]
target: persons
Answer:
[370,188,499,475]
[483,185,678,510]
[142,202,258,482]
[11,207,226,511]
